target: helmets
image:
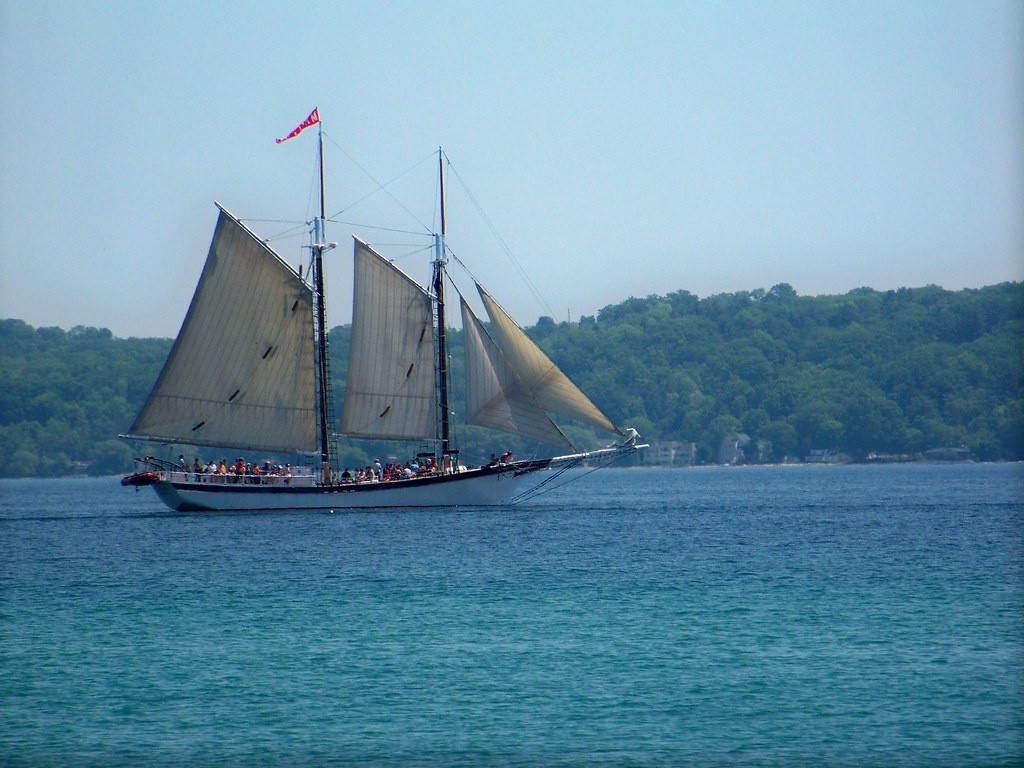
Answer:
[375,459,379,461]
[507,450,512,454]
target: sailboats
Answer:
[115,107,652,511]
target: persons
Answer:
[480,451,513,470]
[179,454,292,485]
[342,451,439,484]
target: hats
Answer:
[265,461,270,464]
[238,456,245,460]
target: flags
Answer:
[275,107,319,144]
